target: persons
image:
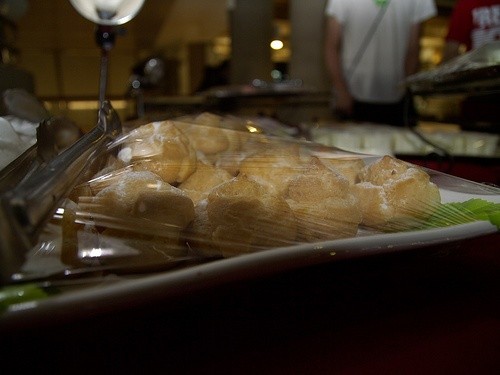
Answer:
[323,1,438,129]
[439,0,500,65]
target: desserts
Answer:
[85,112,441,245]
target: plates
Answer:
[0,162,499,318]
